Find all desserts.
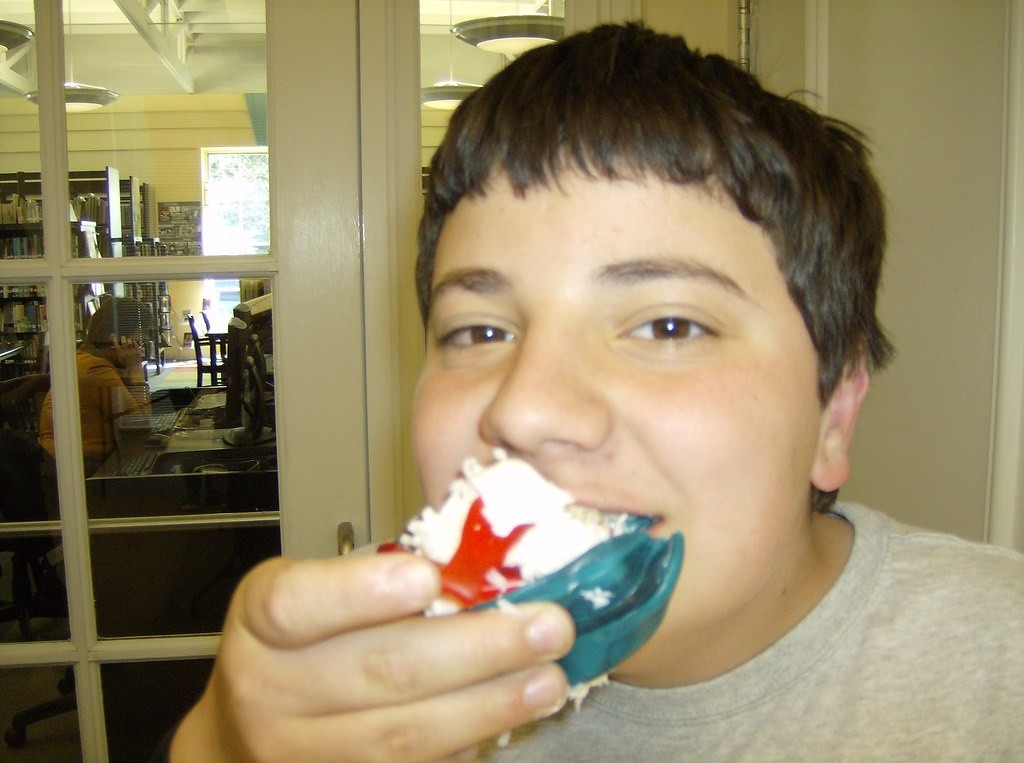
[368,447,685,702]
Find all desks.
[205,325,229,386]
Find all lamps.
[22,0,120,115]
[448,16,563,53]
[420,0,482,110]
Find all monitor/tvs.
[223,293,278,445]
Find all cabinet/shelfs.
[0,167,172,381]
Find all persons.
[20,297,151,599]
[151,21,1024,763]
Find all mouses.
[144,433,168,448]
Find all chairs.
[0,374,237,749]
[188,311,228,387]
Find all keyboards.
[117,453,160,477]
[149,410,183,436]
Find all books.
[0,287,83,331]
[0,234,79,259]
[0,335,38,364]
[0,192,146,229]
